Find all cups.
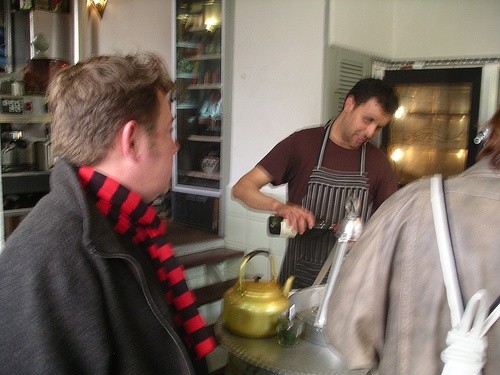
[275,313,304,348]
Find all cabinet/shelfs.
[0,1,227,237]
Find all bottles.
[266,216,341,239]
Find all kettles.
[221,249,294,339]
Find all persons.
[325,109,500,375]
[231,78,399,290]
[0,49,222,375]
